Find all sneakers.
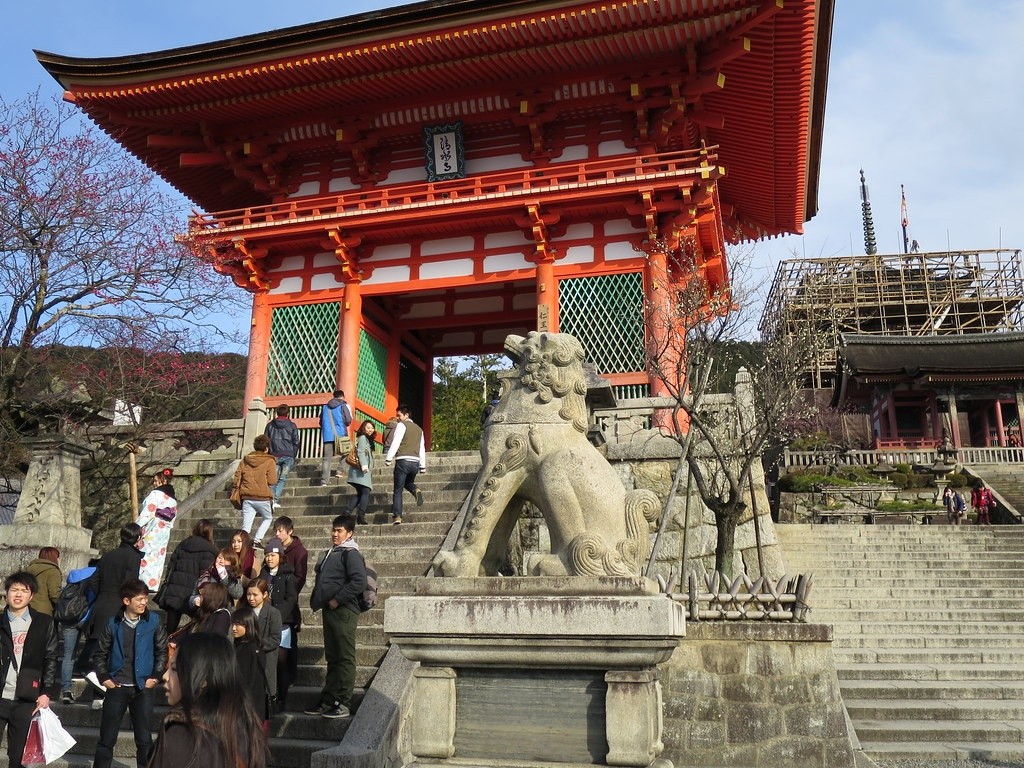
[252,543,265,551]
[304,700,352,718]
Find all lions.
[432,330,663,580]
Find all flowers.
[164,470,170,475]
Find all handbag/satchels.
[345,446,361,469]
[333,436,351,455]
[21,706,78,765]
[16,668,43,700]
[267,695,276,720]
[230,488,243,510]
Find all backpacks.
[341,547,378,611]
[55,580,90,625]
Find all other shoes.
[63,692,76,704]
[85,671,107,710]
[416,489,423,506]
[335,471,344,477]
[357,517,368,525]
[393,516,402,524]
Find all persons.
[135,468,178,593]
[304,515,367,718]
[319,390,352,486]
[146,632,276,768]
[233,404,300,551]
[943,486,963,525]
[0,515,308,768]
[971,482,997,525]
[343,420,377,525]
[382,405,426,524]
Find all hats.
[265,538,284,554]
